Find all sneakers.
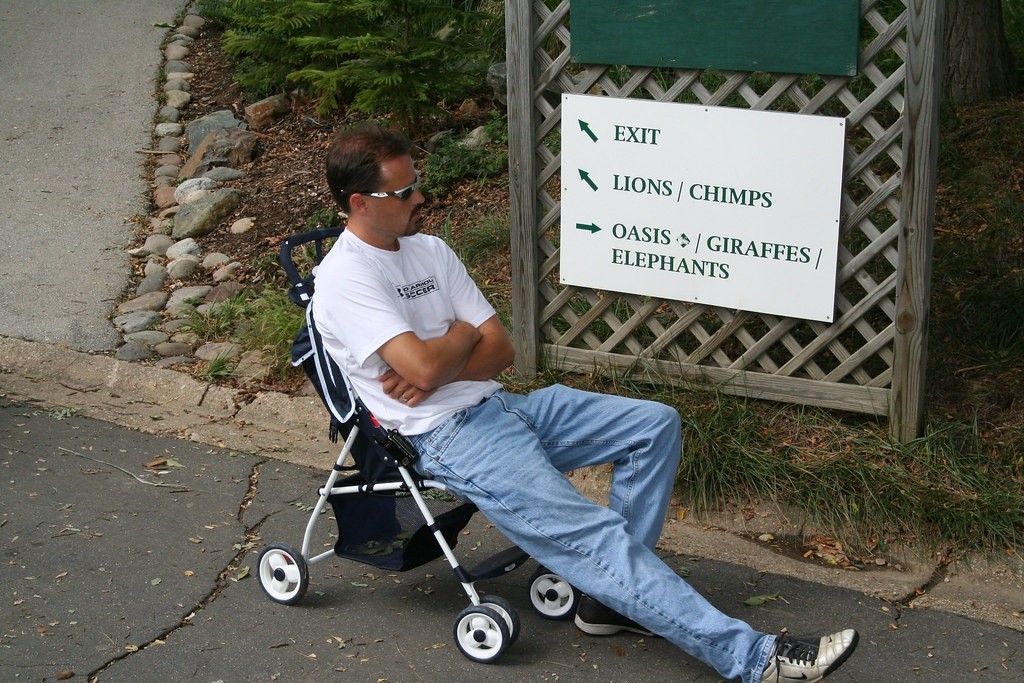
[573,594,656,636]
[759,628,860,683]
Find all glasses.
[355,174,421,202]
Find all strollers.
[257,228,579,663]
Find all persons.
[312,130,859,683]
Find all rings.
[402,395,407,401]
